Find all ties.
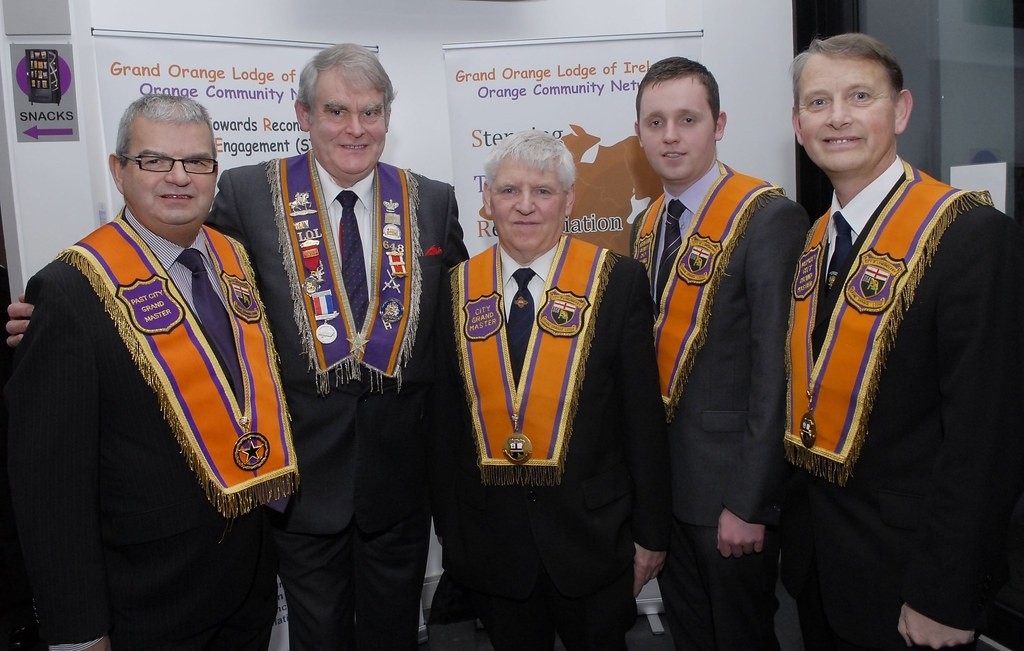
[507,267,534,392]
[815,212,852,297]
[335,190,369,332]
[656,200,686,316]
[176,250,245,414]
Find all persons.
[630,54,809,651]
[780,31,1024,651]
[0,95,299,650]
[6,40,472,651]
[415,130,670,650]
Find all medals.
[233,431,270,471]
[800,413,818,449]
[502,432,534,464]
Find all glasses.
[116,152,218,175]
